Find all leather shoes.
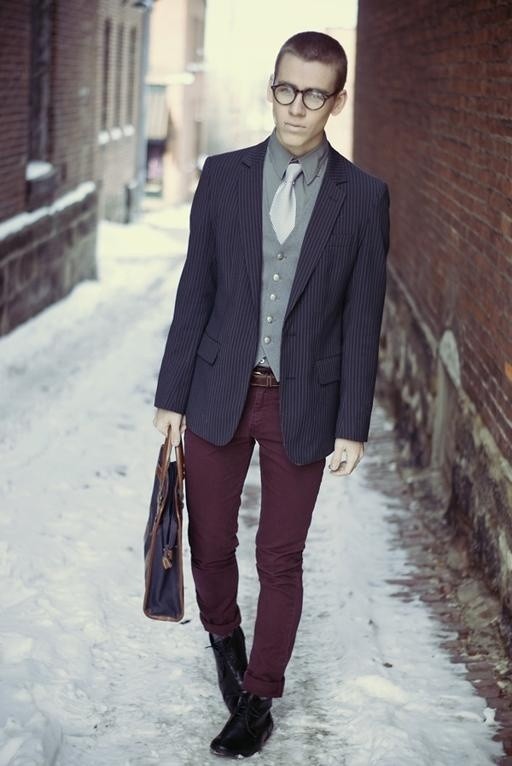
[204,625,248,716]
[209,691,274,759]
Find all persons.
[150,29,392,759]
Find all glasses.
[270,73,340,112]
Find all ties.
[268,163,303,245]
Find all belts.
[253,375,280,388]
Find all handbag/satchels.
[142,424,187,623]
